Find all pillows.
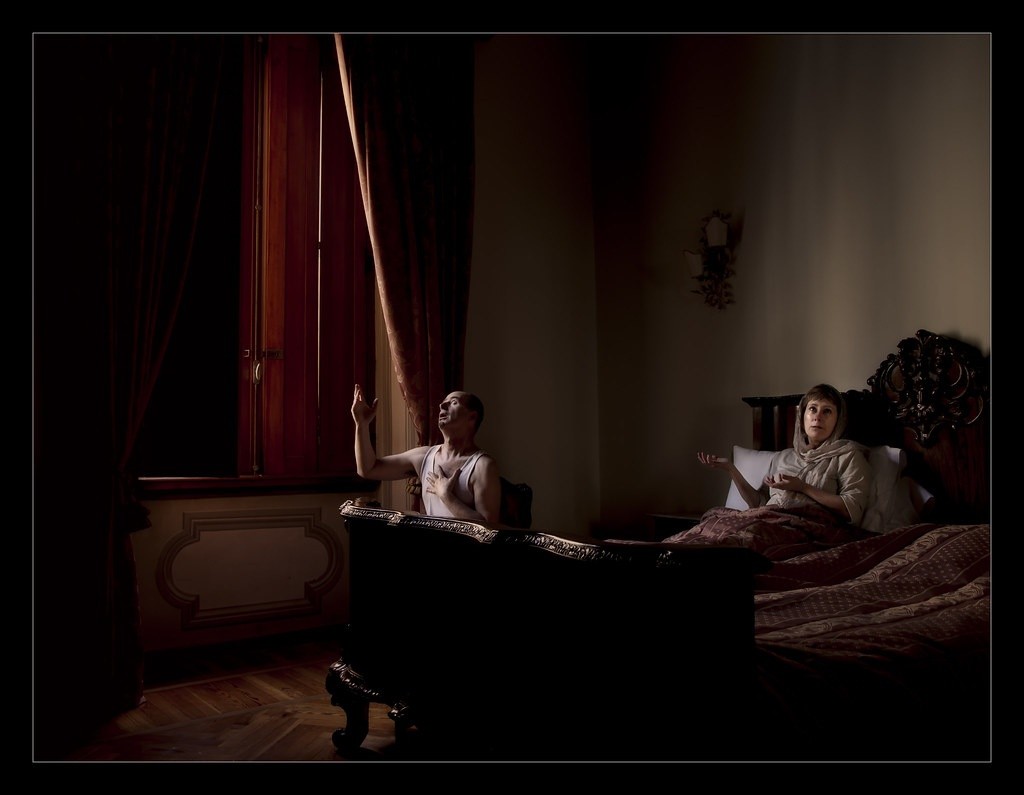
[724,444,936,534]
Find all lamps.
[681,209,734,311]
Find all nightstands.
[588,511,703,543]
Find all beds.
[325,328,990,762]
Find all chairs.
[499,476,533,528]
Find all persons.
[351,383,501,523]
[697,384,872,527]
